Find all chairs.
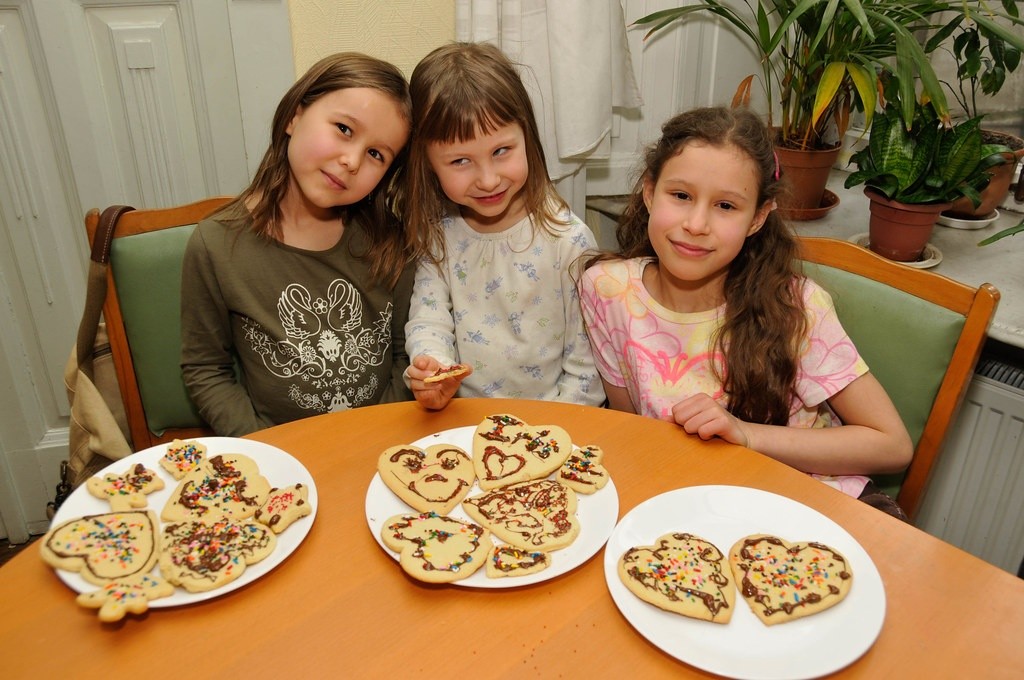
[84,197,240,453]
[797,234,1002,521]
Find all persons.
[400,39,614,407]
[175,50,421,436]
[575,104,917,518]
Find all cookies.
[41,363,853,628]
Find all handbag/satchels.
[45,205,138,527]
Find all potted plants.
[625,0,1024,222]
[844,102,1018,268]
[911,1,1023,230]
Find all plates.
[49,438,318,608]
[365,425,620,589]
[602,484,887,680]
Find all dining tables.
[0,397,1024,680]
